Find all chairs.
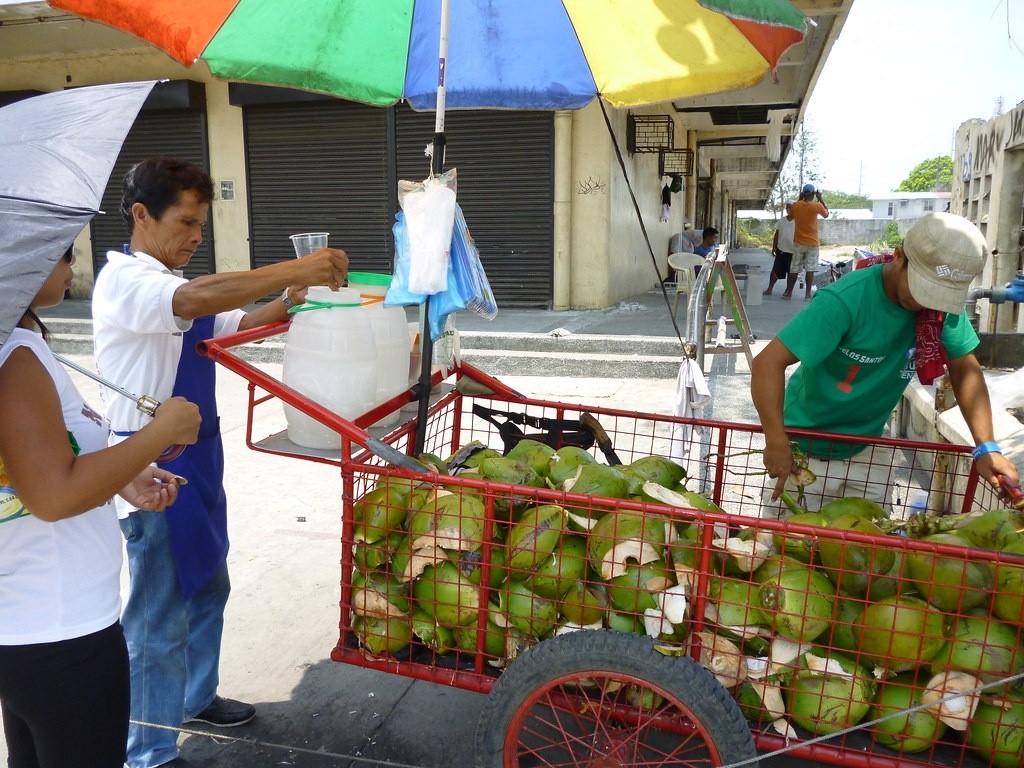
[667,252,725,320]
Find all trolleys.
[195,308,1024,768]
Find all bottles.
[280,285,411,449]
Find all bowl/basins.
[346,271,393,286]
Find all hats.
[803,184,814,194]
[904,210,989,315]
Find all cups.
[288,232,329,259]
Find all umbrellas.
[0,79,187,460]
[45,0,819,460]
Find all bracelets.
[283,287,294,308]
[972,442,1002,461]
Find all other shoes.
[763,290,772,294]
[783,293,791,299]
[805,296,812,301]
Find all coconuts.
[754,496,1024,768]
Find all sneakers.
[158,757,193,768]
[182,696,257,726]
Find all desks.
[733,266,762,306]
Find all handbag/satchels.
[472,404,594,456]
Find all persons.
[0,239,203,768]
[92,158,350,768]
[763,200,795,298]
[784,184,829,302]
[693,227,719,279]
[750,212,1021,549]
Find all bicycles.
[818,259,847,285]
